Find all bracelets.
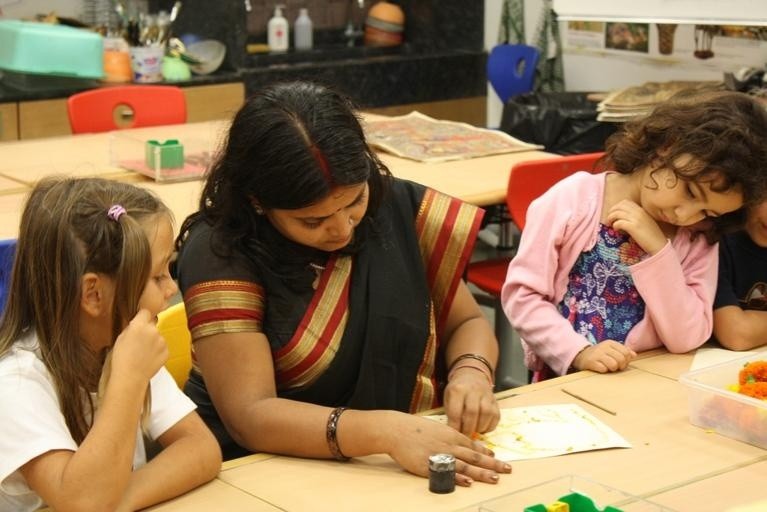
[325,405,352,462]
[447,353,494,376]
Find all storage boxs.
[677,346,767,452]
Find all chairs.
[68,85,187,136]
[155,304,192,390]
[463,152,615,300]
[484,45,540,104]
[0,239,18,319]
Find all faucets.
[342,0,364,46]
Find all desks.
[216,369,767,512]
[123,110,568,250]
[0,133,203,263]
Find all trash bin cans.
[497,87,621,157]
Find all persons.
[0,175,223,512]
[175,78,513,488]
[712,199,767,351]
[500,92,767,382]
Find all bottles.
[293,7,314,50]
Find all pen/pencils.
[562,387,618,415]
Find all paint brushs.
[102,1,183,45]
